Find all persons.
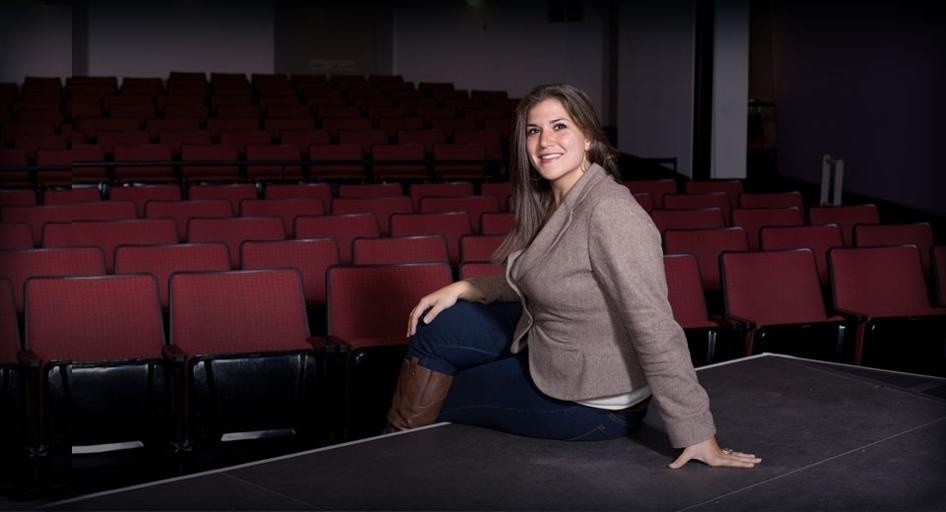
[386,82,762,471]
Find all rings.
[723,446,733,454]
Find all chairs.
[241,239,336,304]
[352,238,448,263]
[830,246,943,367]
[461,261,503,281]
[114,243,231,316]
[662,254,720,366]
[169,270,343,475]
[460,235,510,261]
[760,224,845,280]
[0,277,22,506]
[22,272,169,490]
[327,262,453,440]
[0,246,107,313]
[720,249,847,358]
[853,221,936,275]
[665,229,750,290]
